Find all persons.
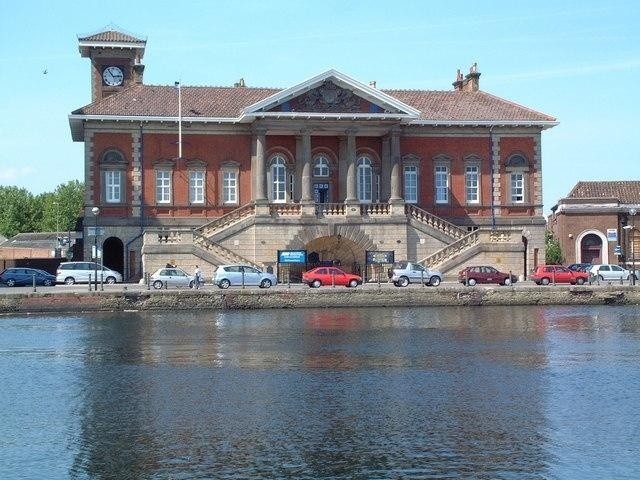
[191,265,201,290]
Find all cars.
[148,266,205,290]
[0,268,56,287]
[457,265,517,286]
[301,266,363,288]
[567,263,638,281]
[211,264,278,289]
[532,265,589,285]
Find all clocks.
[102,66,124,87]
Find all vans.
[56,261,123,285]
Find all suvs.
[388,261,444,287]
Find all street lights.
[91,206,101,290]
[629,209,637,285]
